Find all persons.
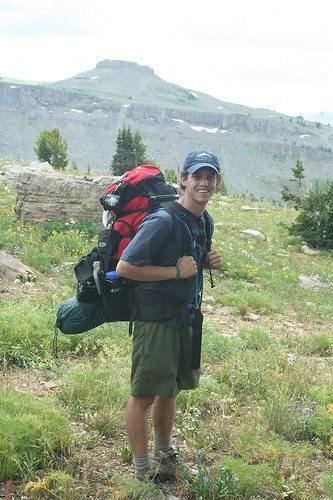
[115,150,222,500]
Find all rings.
[213,259,216,264]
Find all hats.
[183,150,220,175]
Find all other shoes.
[134,451,191,490]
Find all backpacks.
[98,165,180,260]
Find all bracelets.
[175,264,180,282]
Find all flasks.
[105,271,121,287]
